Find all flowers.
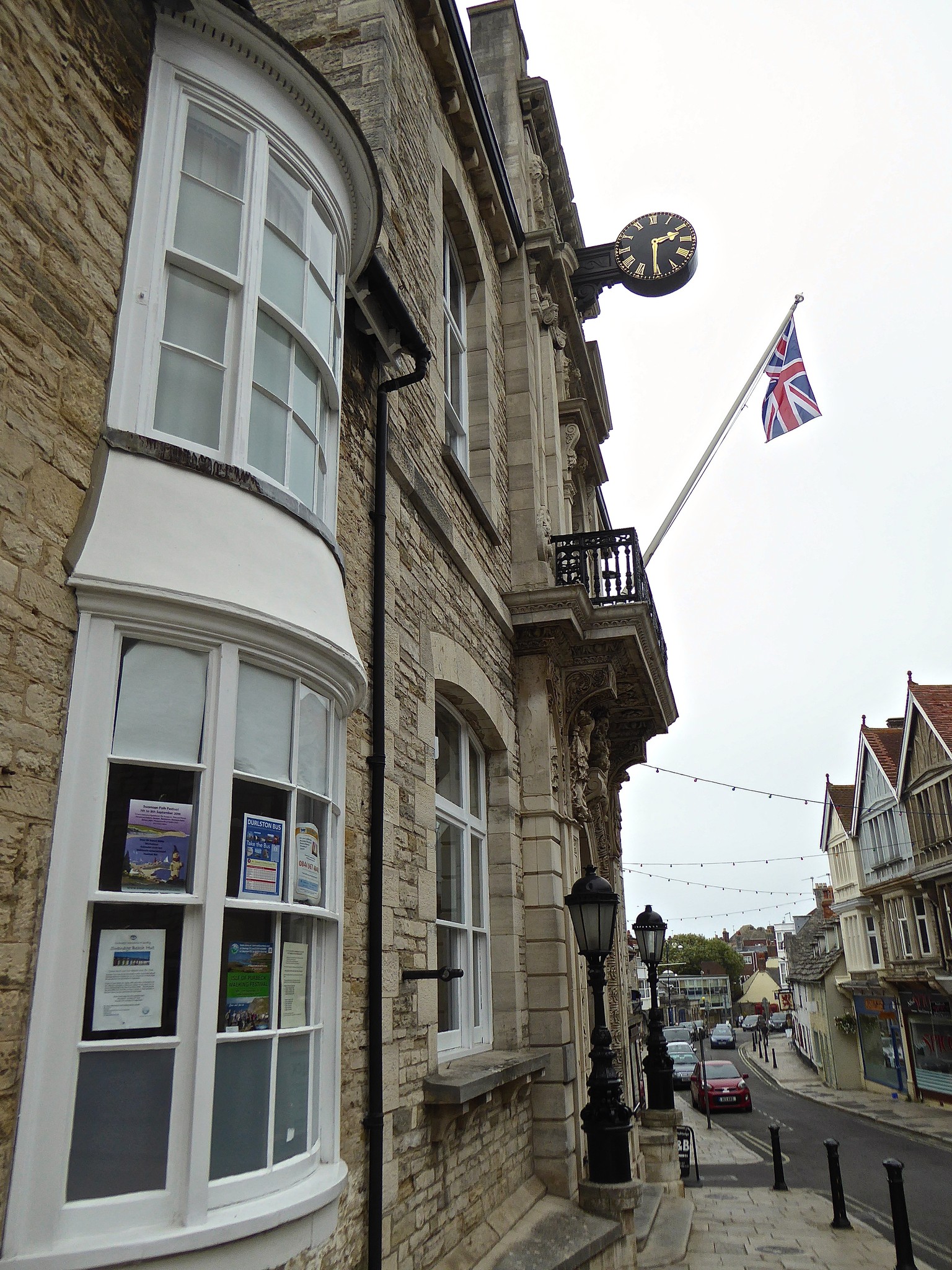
[833,1011,859,1036]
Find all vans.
[742,1014,766,1032]
[769,1013,792,1032]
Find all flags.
[762,317,822,443]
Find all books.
[92,800,321,1031]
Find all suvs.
[678,1021,700,1042]
[709,1023,736,1050]
[662,1025,697,1055]
[693,1020,709,1039]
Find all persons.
[637,1013,743,1051]
[761,1023,769,1046]
[770,1010,774,1020]
[760,1010,767,1024]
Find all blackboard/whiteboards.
[785,1028,792,1038]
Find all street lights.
[631,905,675,1111]
[664,938,683,1027]
[563,862,634,1184]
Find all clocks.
[613,211,700,300]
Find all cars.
[690,1060,752,1115]
[666,1041,697,1060]
[668,1052,703,1086]
